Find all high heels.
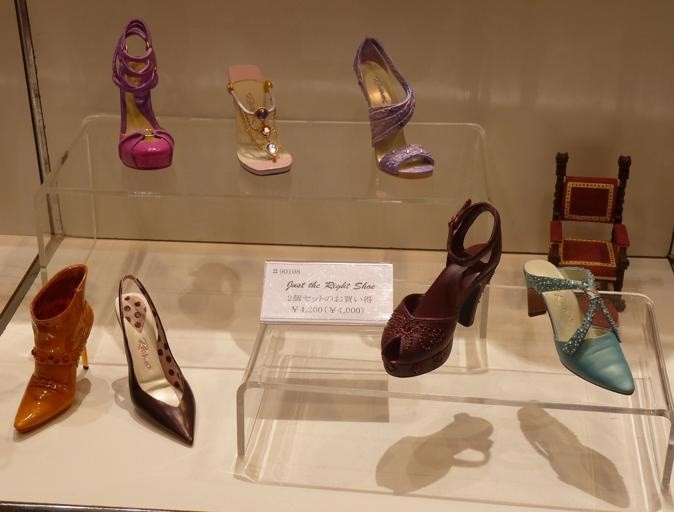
[227,65,292,175]
[112,18,174,169]
[353,36,434,177]
[524,259,635,395]
[13,264,93,432]
[115,275,195,445]
[379,199,502,378]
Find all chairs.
[545,151,631,312]
